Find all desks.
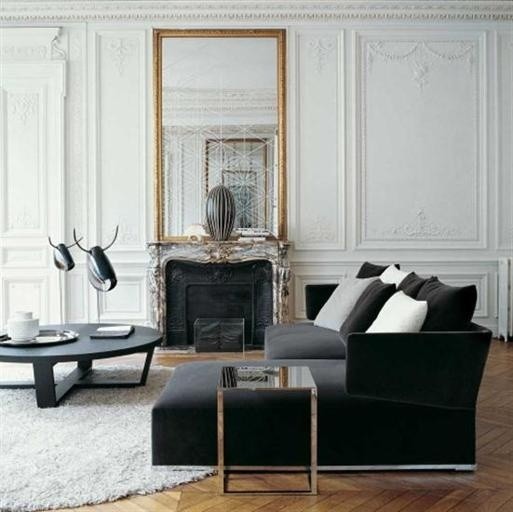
[214,365,318,496]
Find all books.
[238,230,270,240]
[89,326,133,339]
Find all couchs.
[150,321,493,474]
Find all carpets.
[0,365,217,512]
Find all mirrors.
[152,28,288,244]
[205,138,267,229]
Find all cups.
[13,311,32,319]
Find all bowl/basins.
[6,319,39,342]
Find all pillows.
[367,289,428,334]
[399,272,437,297]
[314,278,378,331]
[419,275,477,331]
[339,278,396,346]
[356,260,400,278]
[378,265,411,288]
